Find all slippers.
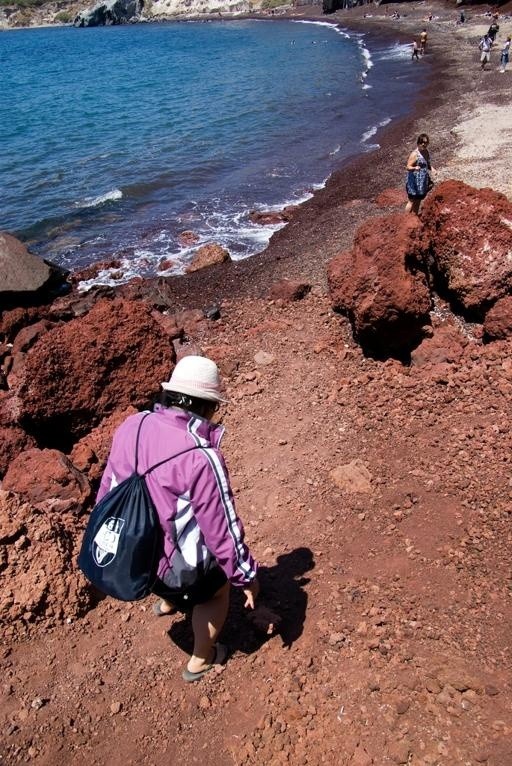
[182,643,229,684]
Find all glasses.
[420,141,429,144]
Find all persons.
[405,133,433,215]
[419,29,427,54]
[412,40,419,61]
[496,37,511,73]
[478,35,492,71]
[96,355,260,683]
[487,19,499,47]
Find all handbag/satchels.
[407,170,432,199]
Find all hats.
[160,354,231,408]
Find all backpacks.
[77,409,215,602]
